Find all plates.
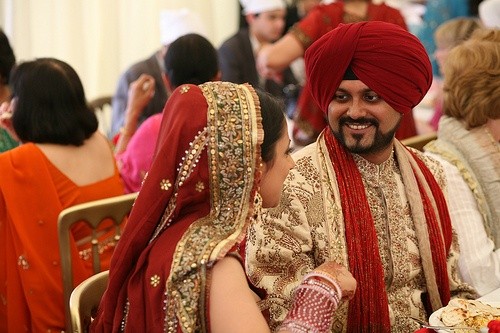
[429,307,492,333]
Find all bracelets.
[301,270,341,307]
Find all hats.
[239,0,286,14]
[304,19,433,114]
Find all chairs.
[57,190,141,333]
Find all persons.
[87,81,358,332]
[111,34,221,131]
[259,0,410,70]
[0,27,20,154]
[244,22,500,333]
[216,1,302,117]
[427,16,485,132]
[422,27,500,248]
[109,35,222,191]
[0,57,137,333]
